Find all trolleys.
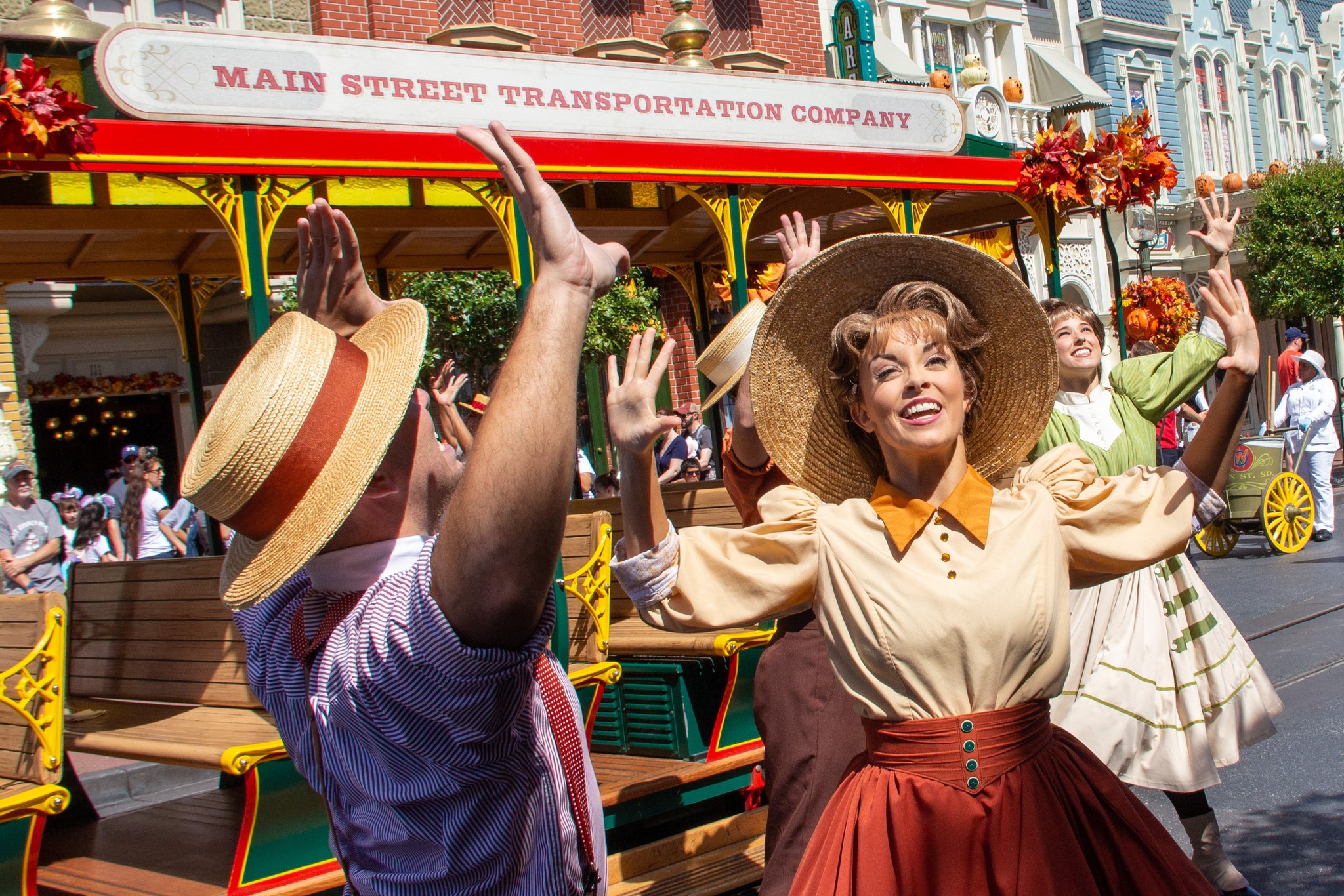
[1193,424,1314,559]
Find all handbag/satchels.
[1156,444,1164,466]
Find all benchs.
[0,480,779,896]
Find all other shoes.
[1331,476,1344,486]
[1299,533,1314,541]
[1222,886,1261,896]
[1314,529,1332,541]
[1190,558,1200,572]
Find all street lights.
[1117,191,1173,290]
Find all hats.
[695,297,768,412]
[1290,349,1327,377]
[179,298,429,612]
[459,393,491,415]
[121,445,140,460]
[1284,327,1308,342]
[2,462,33,482]
[749,231,1059,504]
[686,436,699,458]
[674,402,698,414]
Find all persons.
[695,207,866,896]
[1175,391,1212,450]
[0,463,69,599]
[50,442,234,584]
[569,445,596,501]
[672,402,717,482]
[1019,193,1284,896]
[427,358,493,463]
[1275,327,1344,486]
[183,120,627,896]
[1127,340,1201,575]
[655,407,688,484]
[594,469,620,497]
[1259,348,1340,542]
[680,458,702,482]
[604,219,1264,896]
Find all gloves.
[1259,426,1267,436]
[1298,418,1312,433]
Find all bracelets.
[24,581,35,591]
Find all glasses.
[125,455,138,463]
[147,467,164,473]
[686,470,700,476]
[677,413,690,418]
